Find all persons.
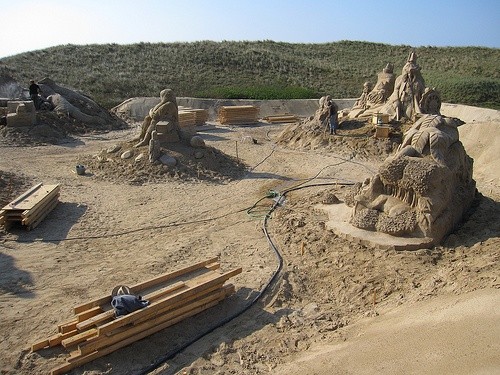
[382,53,428,122]
[132,90,181,148]
[28,81,43,110]
[149,131,160,162]
[326,103,338,135]
[368,61,396,105]
